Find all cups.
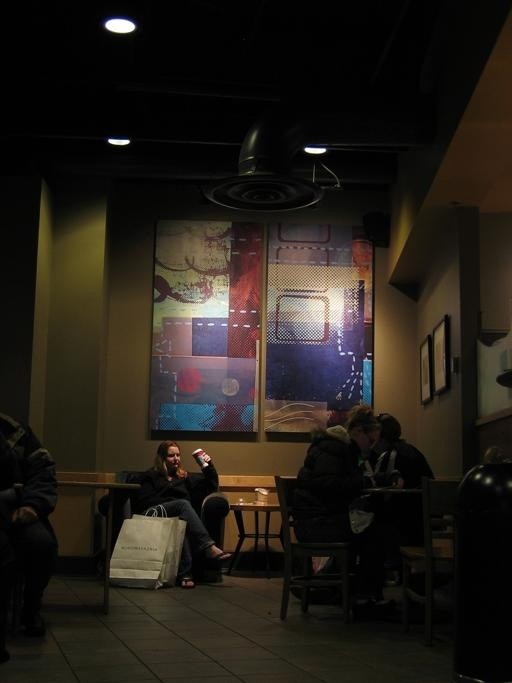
[191,449,209,470]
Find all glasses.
[365,433,375,447]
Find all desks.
[11,474,142,621]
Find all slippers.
[204,552,234,562]
[181,579,195,589]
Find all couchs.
[97,469,228,585]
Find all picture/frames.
[411,314,455,405]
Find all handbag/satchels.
[109,504,188,590]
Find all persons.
[289,404,441,618]
[134,438,233,589]
[0,408,60,648]
